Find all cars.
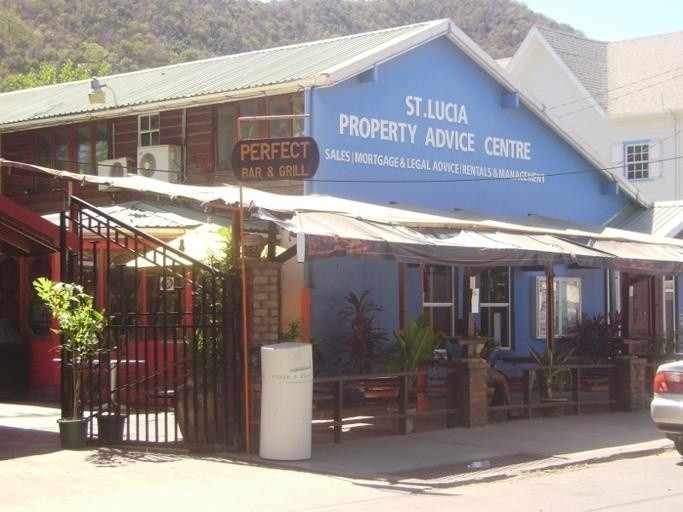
[648,359,683,456]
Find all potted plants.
[386,309,442,435]
[33,276,114,449]
[521,338,575,417]
[96,306,127,442]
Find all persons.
[462,328,518,419]
